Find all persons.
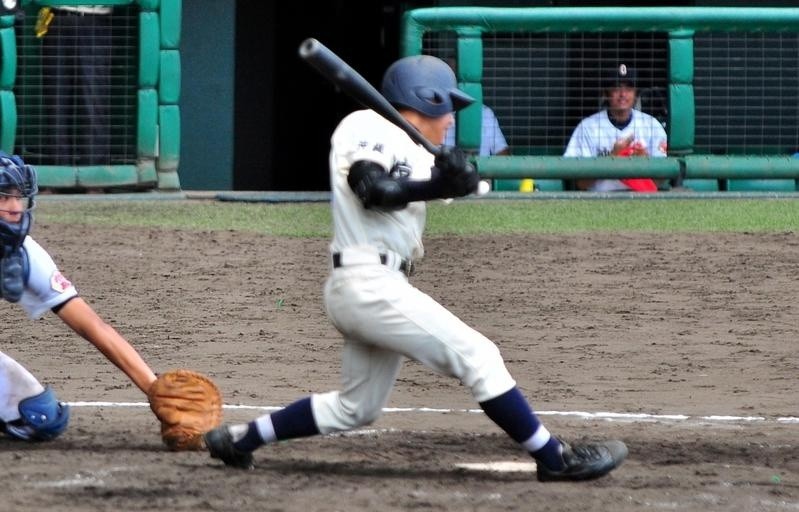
[38,4,114,194]
[443,55,511,156]
[563,63,667,191]
[202,54,628,481]
[0,155,222,449]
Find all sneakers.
[203,426,255,471]
[536,440,629,482]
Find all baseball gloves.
[147,370,223,451]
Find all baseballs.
[474,179,490,196]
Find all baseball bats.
[298,37,491,199]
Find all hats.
[603,63,637,89]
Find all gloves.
[434,145,479,198]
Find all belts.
[333,251,414,276]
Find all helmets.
[0,154,37,213]
[381,54,477,117]
